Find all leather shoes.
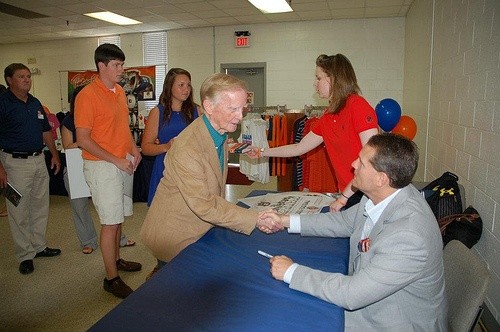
[19,260,34,275]
[35,246,61,257]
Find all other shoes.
[117,259,142,272]
[83,246,93,254]
[125,239,135,246]
[103,276,134,299]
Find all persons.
[246,54,380,212]
[140,73,284,267]
[259,133,448,332]
[73,43,143,300]
[61,86,136,254]
[142,67,201,205]
[0,63,61,274]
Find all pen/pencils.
[258,250,273,259]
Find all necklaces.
[175,106,180,115]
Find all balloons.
[392,115,417,141]
[374,98,401,133]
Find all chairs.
[443,240,490,332]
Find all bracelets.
[259,146,265,159]
[341,192,350,200]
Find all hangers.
[245,105,328,119]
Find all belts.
[0,148,43,156]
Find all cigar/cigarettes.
[258,249,275,260]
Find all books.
[0,181,23,208]
[227,141,251,154]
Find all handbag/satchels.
[418,171,462,219]
[437,206,483,250]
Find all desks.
[42,148,155,203]
[87,191,350,332]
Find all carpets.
[226,168,256,186]
